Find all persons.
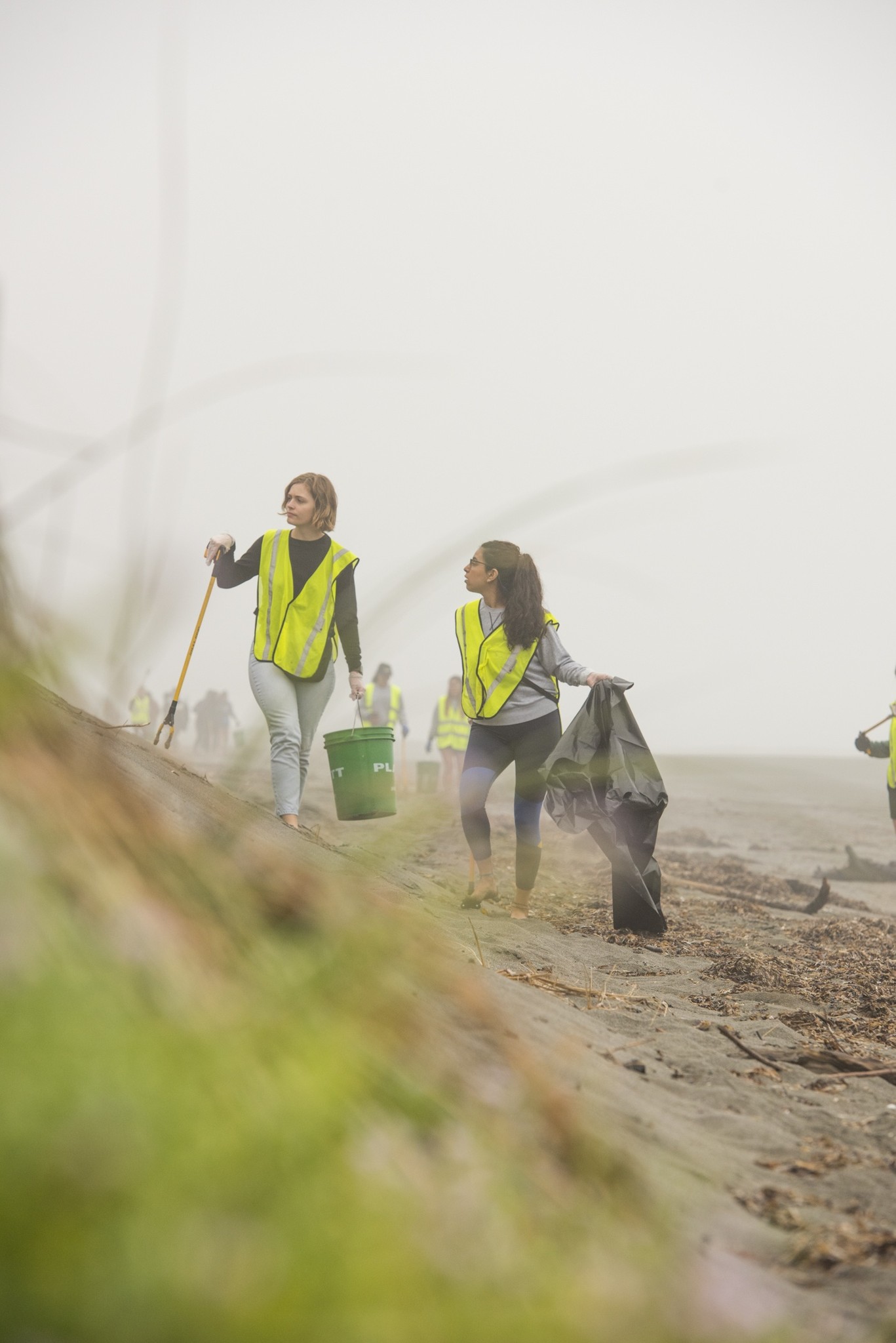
[424,674,472,813]
[453,537,615,923]
[354,664,409,738]
[205,472,367,834]
[854,664,896,824]
[130,684,241,766]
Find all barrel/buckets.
[417,754,441,791]
[232,723,246,748]
[323,693,397,821]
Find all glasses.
[469,558,487,568]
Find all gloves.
[854,735,870,751]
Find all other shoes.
[461,871,499,908]
